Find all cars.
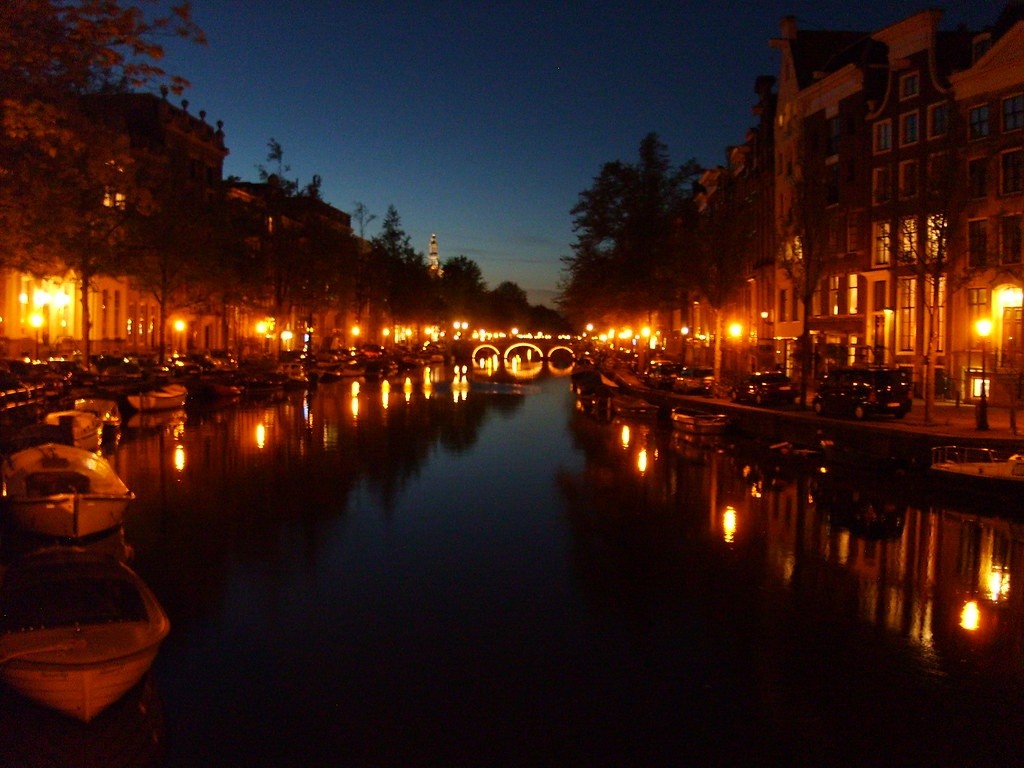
[643,361,677,387]
[673,366,714,394]
[728,371,796,408]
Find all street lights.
[975,317,991,430]
[32,314,41,360]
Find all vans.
[813,365,911,418]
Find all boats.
[1,541,170,722]
[34,395,124,454]
[570,351,618,393]
[189,350,455,403]
[0,442,170,544]
[925,445,1024,512]
[610,391,659,420]
[669,405,727,434]
[128,381,188,410]
